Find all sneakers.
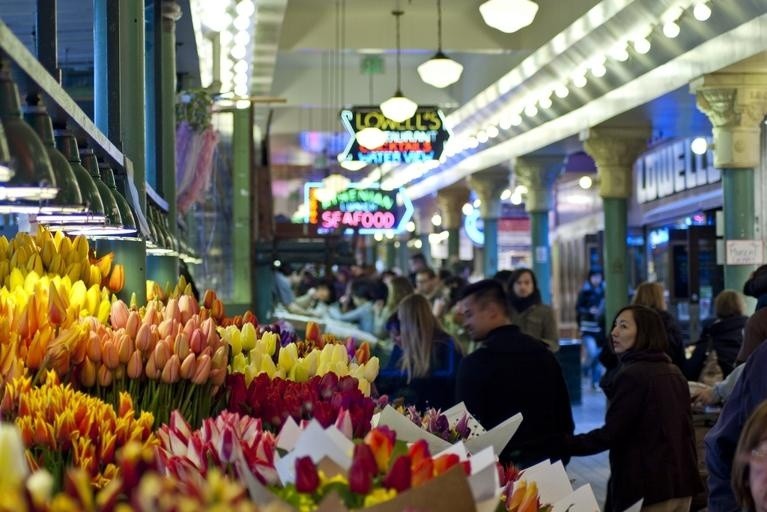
[582,365,602,394]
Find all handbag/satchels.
[699,347,725,387]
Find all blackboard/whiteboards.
[584,242,603,282]
[624,245,648,289]
[668,240,690,305]
[688,224,724,287]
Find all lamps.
[0,54,202,266]
[336,0,541,170]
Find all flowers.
[0,223,573,510]
